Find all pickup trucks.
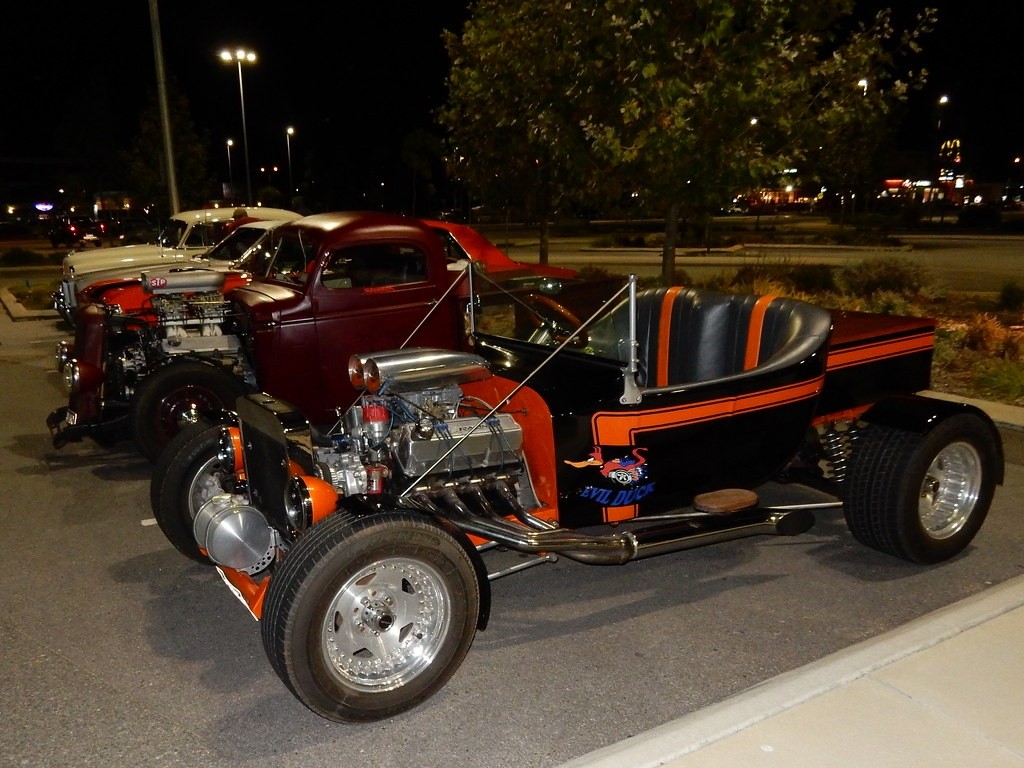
[42,206,625,467]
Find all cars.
[40,211,107,249]
[45,205,581,341]
[145,256,1007,726]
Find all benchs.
[609,286,835,412]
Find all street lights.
[226,139,235,205]
[219,47,259,206]
[285,126,296,207]
